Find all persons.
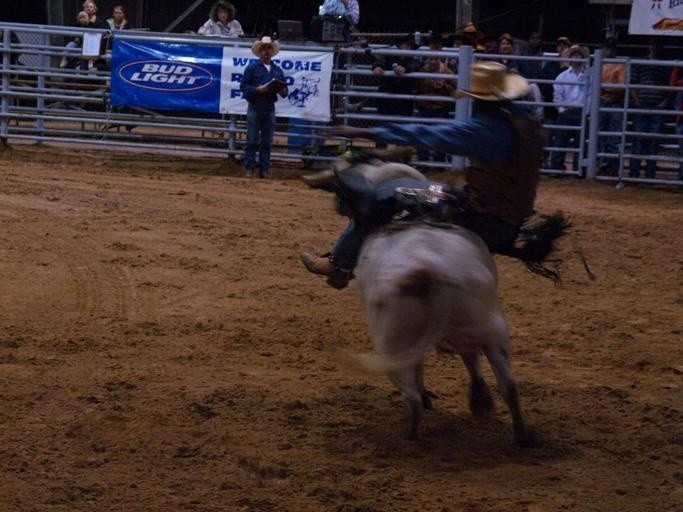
[372,21,683,191]
[301,61,546,289]
[60,1,136,114]
[199,2,243,38]
[1,30,22,151]
[320,1,359,24]
[241,36,288,180]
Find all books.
[264,78,286,96]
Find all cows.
[295,144,533,463]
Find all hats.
[250,36,281,57]
[560,44,590,67]
[497,33,513,47]
[556,37,572,46]
[455,21,485,40]
[452,61,531,102]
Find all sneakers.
[259,170,273,180]
[244,169,253,177]
[300,250,355,290]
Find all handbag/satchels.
[303,14,352,43]
